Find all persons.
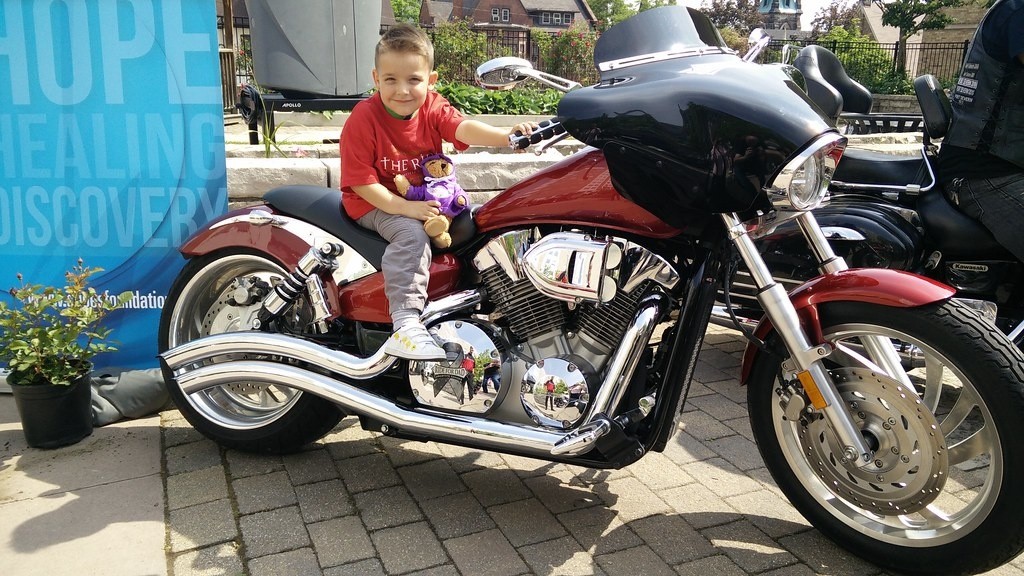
[939,0,1024,264]
[340,25,539,362]
[460,346,474,404]
[482,359,500,393]
[735,136,766,186]
[544,376,555,411]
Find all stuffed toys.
[394,154,468,247]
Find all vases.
[6,360,94,452]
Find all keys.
[951,190,959,205]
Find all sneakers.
[385,323,447,361]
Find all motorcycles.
[155,2,1024,576]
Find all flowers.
[0,256,133,386]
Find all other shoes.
[552,409,555,411]
[545,408,547,410]
